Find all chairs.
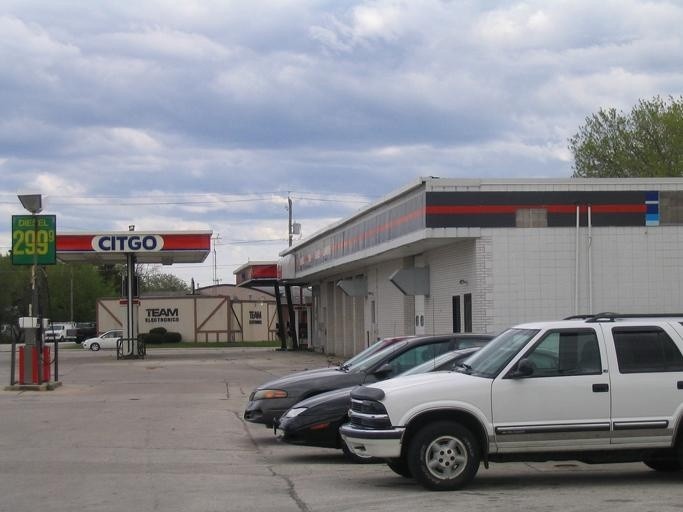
[576,340,600,374]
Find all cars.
[44,321,124,351]
